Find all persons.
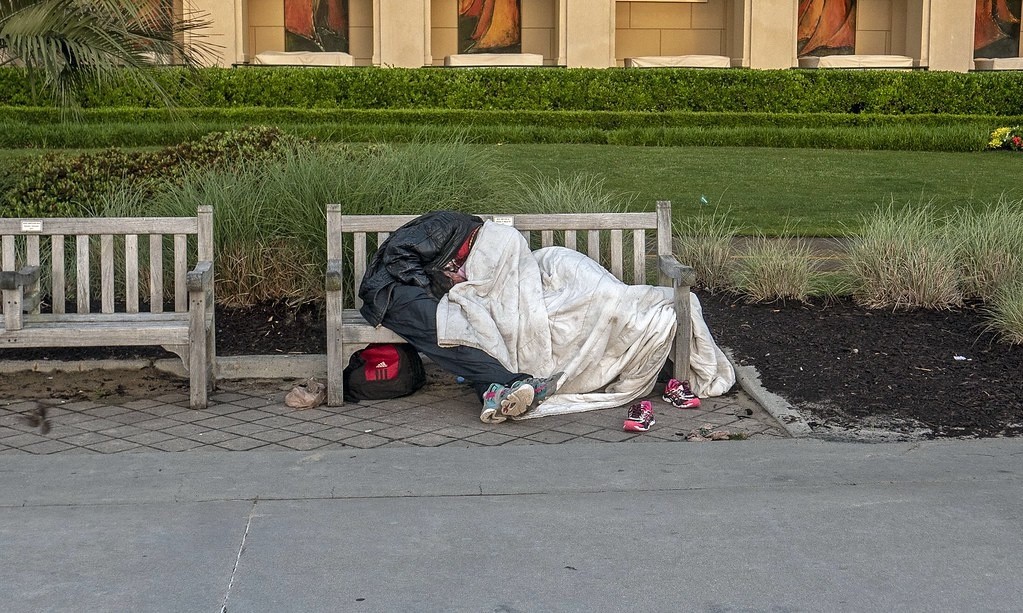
[359,208,570,423]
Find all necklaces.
[469,224,484,251]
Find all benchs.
[324,195,697,407]
[0,207,222,408]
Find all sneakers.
[479,382,534,424]
[622,400,657,431]
[510,371,570,421]
[663,378,703,410]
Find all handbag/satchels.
[342,342,427,404]
